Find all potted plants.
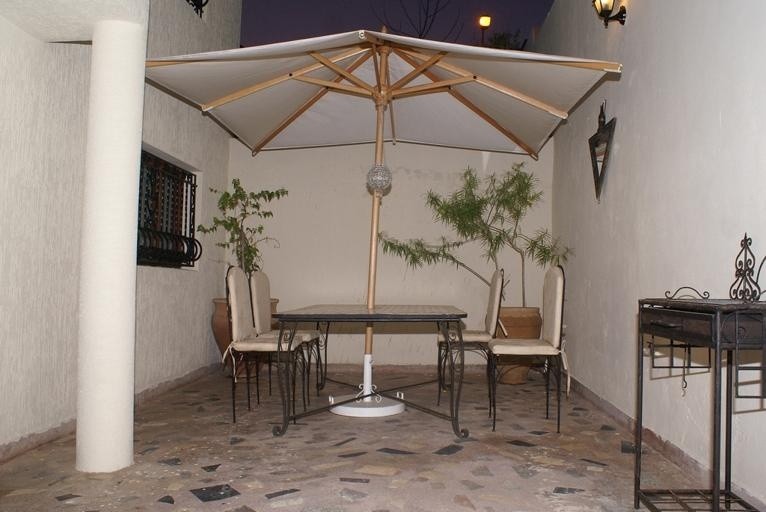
[375,163,576,385]
[196,178,290,378]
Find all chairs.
[486,264,566,434]
[437,268,505,406]
[226,265,322,426]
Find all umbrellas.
[143,25,622,403]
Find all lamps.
[592,0,626,26]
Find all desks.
[271,302,469,439]
[633,231,766,511]
